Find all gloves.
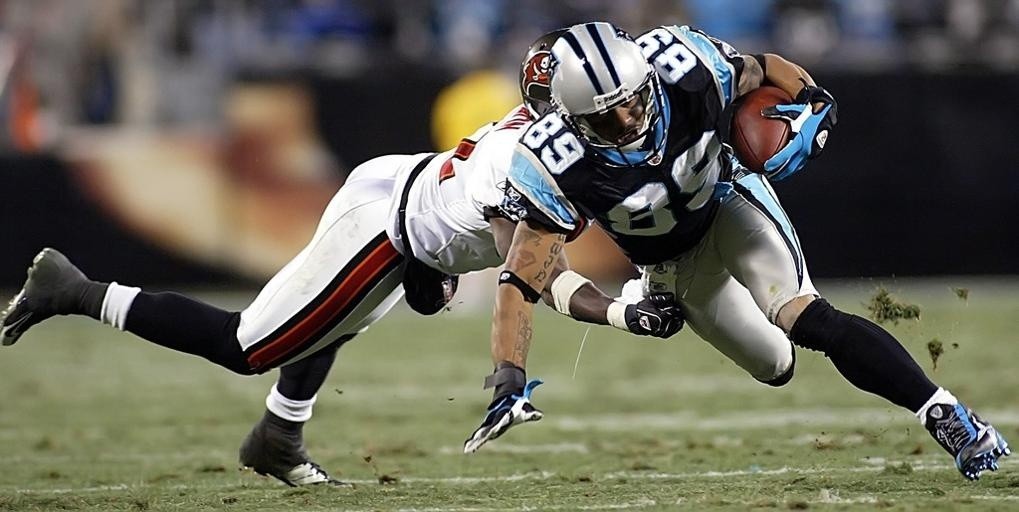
[759,76,840,184]
[624,290,686,341]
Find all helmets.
[517,19,669,173]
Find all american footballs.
[733,86,793,174]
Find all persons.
[0,23,686,484]
[455,14,1012,485]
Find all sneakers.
[0,244,114,349]
[237,405,334,490]
[923,400,1013,484]
[460,360,545,456]
